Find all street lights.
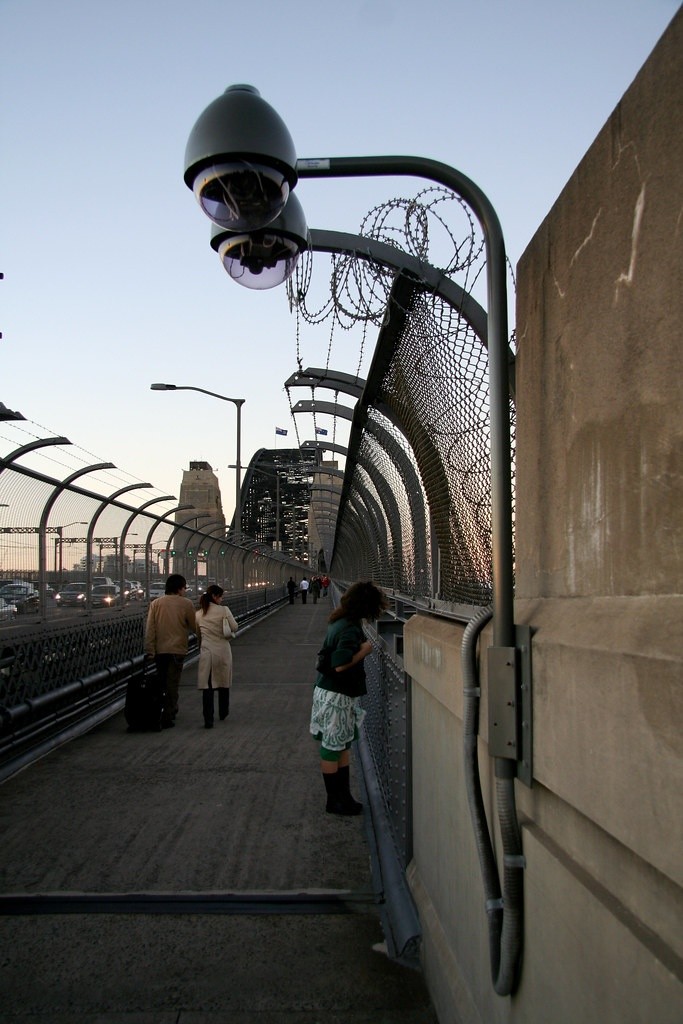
[150,540,169,576]
[257,499,296,558]
[228,465,280,553]
[150,382,245,539]
[59,521,89,570]
[114,533,138,578]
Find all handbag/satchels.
[223,605,235,641]
[315,624,365,684]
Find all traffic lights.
[171,549,225,566]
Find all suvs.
[92,576,114,586]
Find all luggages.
[123,653,164,732]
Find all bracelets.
[355,652,364,660]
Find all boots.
[322,765,364,816]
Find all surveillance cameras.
[184,84,300,233]
[210,191,307,291]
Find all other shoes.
[161,703,180,729]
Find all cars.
[55,583,93,607]
[0,584,40,616]
[109,578,166,602]
[32,582,55,600]
[81,584,121,609]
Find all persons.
[143,574,195,730]
[300,577,309,604]
[195,585,238,729]
[309,574,330,604]
[287,577,296,604]
[309,580,387,816]
